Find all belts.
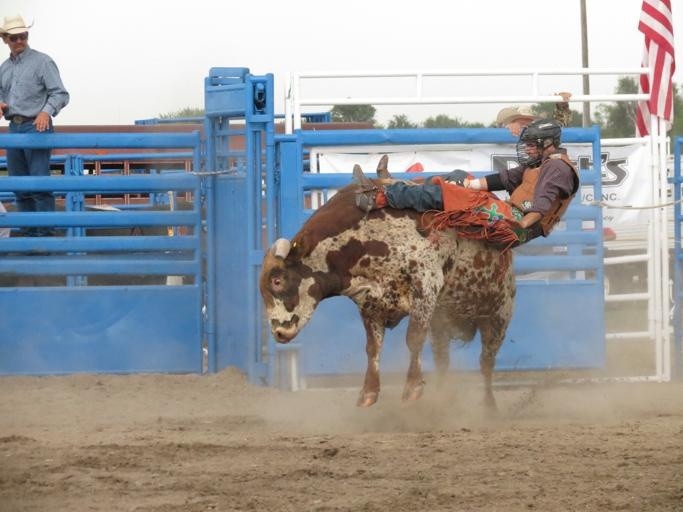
[9,115,34,125]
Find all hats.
[0,13,36,35]
[495,105,537,126]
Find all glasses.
[8,34,27,42]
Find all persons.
[496,109,541,139]
[539,89,581,128]
[350,117,579,255]
[0,10,69,260]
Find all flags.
[636,1,677,150]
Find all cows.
[259,177,516,412]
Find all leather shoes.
[375,154,392,179]
[351,163,379,213]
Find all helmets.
[523,118,563,149]
